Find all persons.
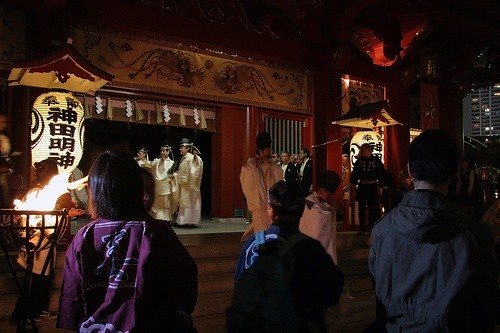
[445,148,480,201]
[130,139,207,230]
[239,132,345,264]
[228,182,344,332]
[341,143,388,235]
[369,130,499,332]
[0,114,15,210]
[12,158,86,331]
[57,150,198,333]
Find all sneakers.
[9,313,32,324]
[34,310,57,321]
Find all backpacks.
[227,230,305,333]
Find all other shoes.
[368,231,372,235]
[356,230,364,234]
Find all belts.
[360,179,377,183]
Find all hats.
[318,170,341,193]
[161,144,170,149]
[257,133,271,149]
[180,137,192,146]
